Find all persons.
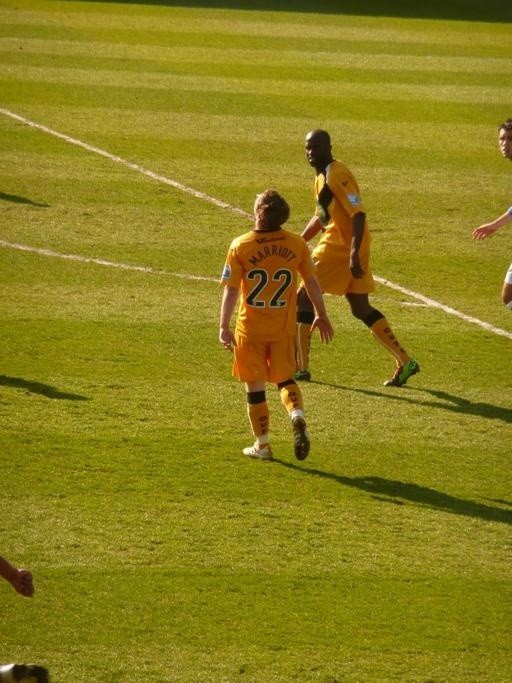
[0,556,52,683]
[218,189,334,461]
[471,118,512,310]
[296,128,420,386]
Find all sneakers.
[383,357,420,387]
[243,443,274,460]
[0,662,49,682]
[295,370,311,382]
[292,416,310,460]
